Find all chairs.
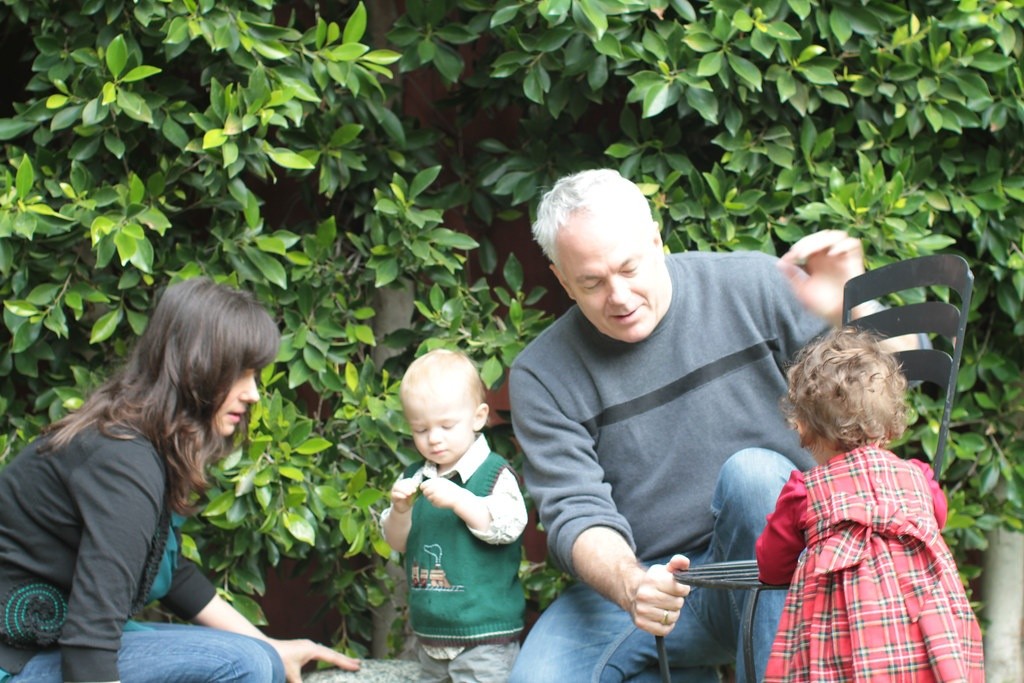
[643,251,980,683]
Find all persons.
[756,327,985,683]
[379,349,528,683]
[507,169,933,683]
[0,279,361,683]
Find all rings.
[662,610,670,625]
